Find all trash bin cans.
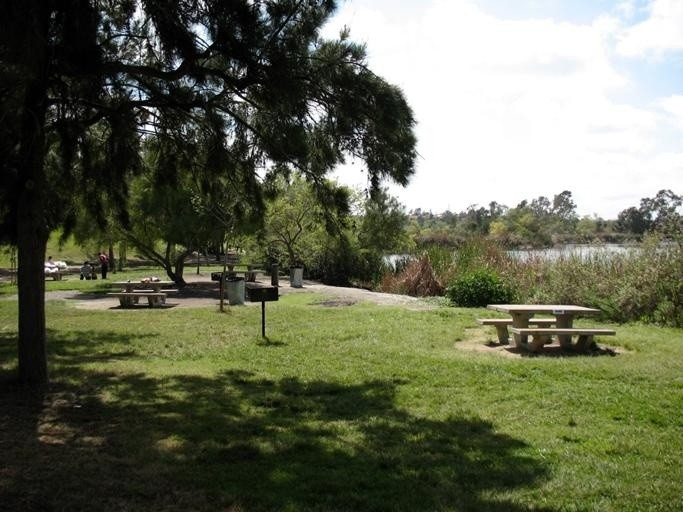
[290,265,304,288]
[225,276,245,304]
[271,264,279,286]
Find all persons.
[90,264,96,280]
[78,261,91,279]
[47,256,53,265]
[97,252,106,279]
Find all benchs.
[216,270,266,282]
[106,289,178,307]
[475,318,616,355]
[44,271,71,281]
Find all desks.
[486,304,601,349]
[45,267,65,280]
[220,264,264,281]
[111,281,175,307]
